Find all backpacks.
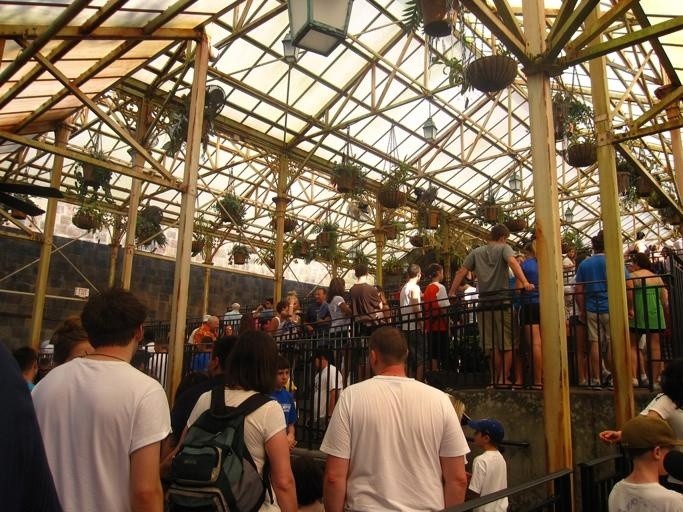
[165,382,277,512]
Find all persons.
[303,265,391,385]
[441,386,471,426]
[399,224,536,390]
[292,453,325,509]
[3,287,173,510]
[608,415,681,509]
[514,233,681,391]
[188,291,302,351]
[313,346,344,446]
[599,359,681,493]
[470,416,509,509]
[161,330,298,509]
[319,325,471,509]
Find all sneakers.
[602,371,611,384]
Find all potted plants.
[550,68,598,166]
[499,199,527,232]
[443,16,517,93]
[72,107,214,255]
[561,229,583,252]
[403,0,463,37]
[616,144,681,236]
[217,127,496,283]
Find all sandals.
[638,378,649,388]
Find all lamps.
[423,38,438,142]
[286,2,352,56]
[565,201,573,223]
[282,30,298,63]
[509,153,521,192]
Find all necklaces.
[81,350,125,361]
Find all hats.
[622,415,683,449]
[468,418,504,443]
[203,315,210,322]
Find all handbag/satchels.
[282,321,293,332]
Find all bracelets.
[328,416,331,418]
[382,303,388,305]
